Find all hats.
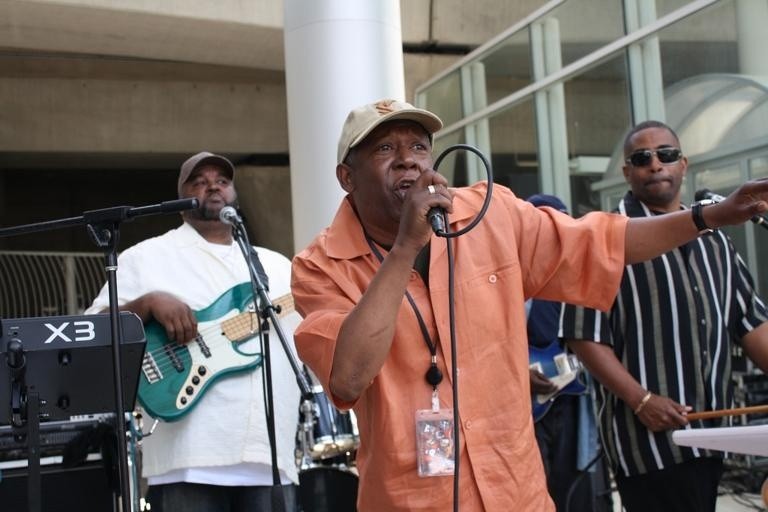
[335,97,444,168]
[525,192,570,215]
[176,150,236,196]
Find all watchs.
[691,198,720,236]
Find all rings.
[428,185,436,195]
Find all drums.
[300,384,361,462]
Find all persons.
[523,193,613,512]
[290,101,768,512]
[84,152,304,512]
[555,120,768,512]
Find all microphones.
[218,205,242,227]
[426,204,444,237]
[693,188,768,228]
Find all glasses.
[623,143,685,168]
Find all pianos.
[0,312,146,416]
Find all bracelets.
[633,391,651,415]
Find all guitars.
[529,341,593,424]
[134,282,295,422]
